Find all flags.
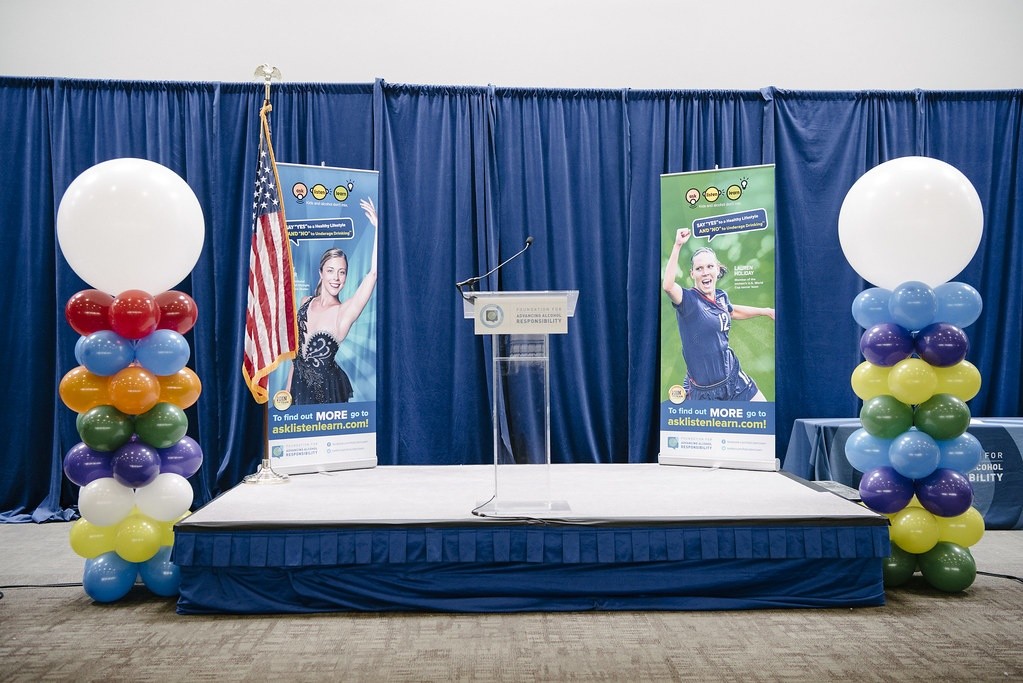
[241,103,298,405]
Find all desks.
[781,418,1023,530]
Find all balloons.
[57,159,205,297]
[845,427,982,478]
[882,540,976,592]
[851,282,983,334]
[859,323,969,368]
[859,468,973,517]
[75,405,188,451]
[851,359,982,406]
[859,494,984,553]
[77,473,194,525]
[74,330,190,376]
[60,363,202,414]
[67,291,198,336]
[70,509,193,601]
[63,436,203,489]
[860,394,971,441]
[838,157,983,291]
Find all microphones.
[455,237,534,288]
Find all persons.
[661,228,776,402]
[284,197,377,403]
